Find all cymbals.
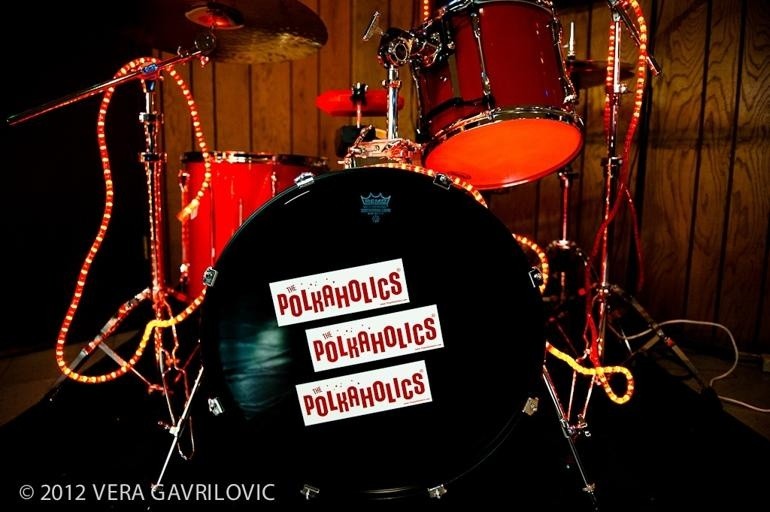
[561,61,635,89]
[148,0,328,64]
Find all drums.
[411,2,585,194]
[180,151,329,304]
[344,138,423,166]
[202,164,546,503]
